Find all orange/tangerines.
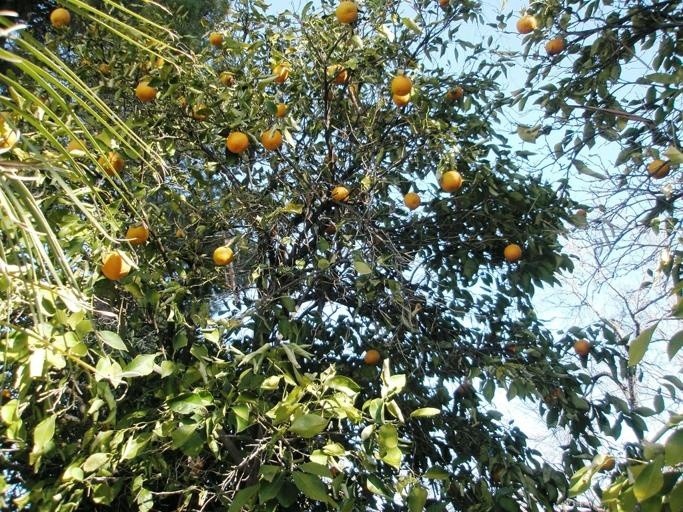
[0,1,671,472]
